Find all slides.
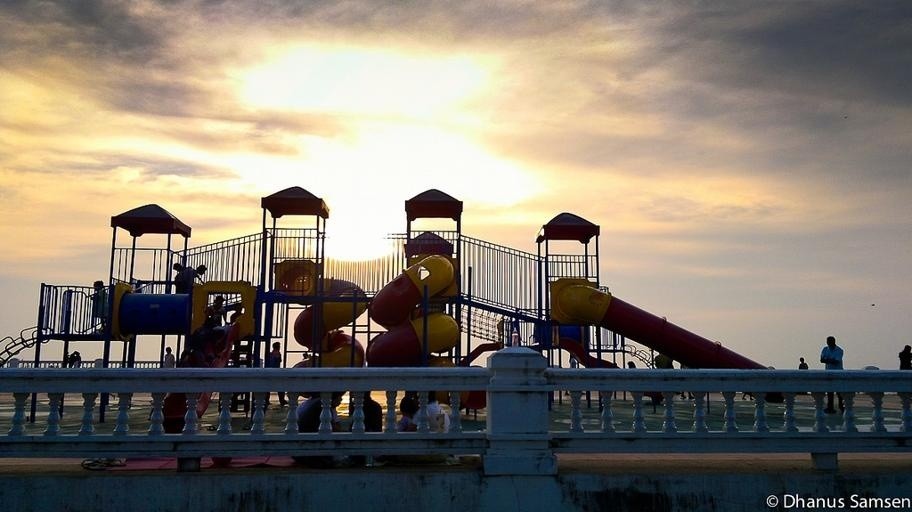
[163,323,238,425]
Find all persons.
[742,393,754,400]
[799,358,808,395]
[820,337,844,414]
[565,353,585,395]
[680,364,694,399]
[899,345,912,370]
